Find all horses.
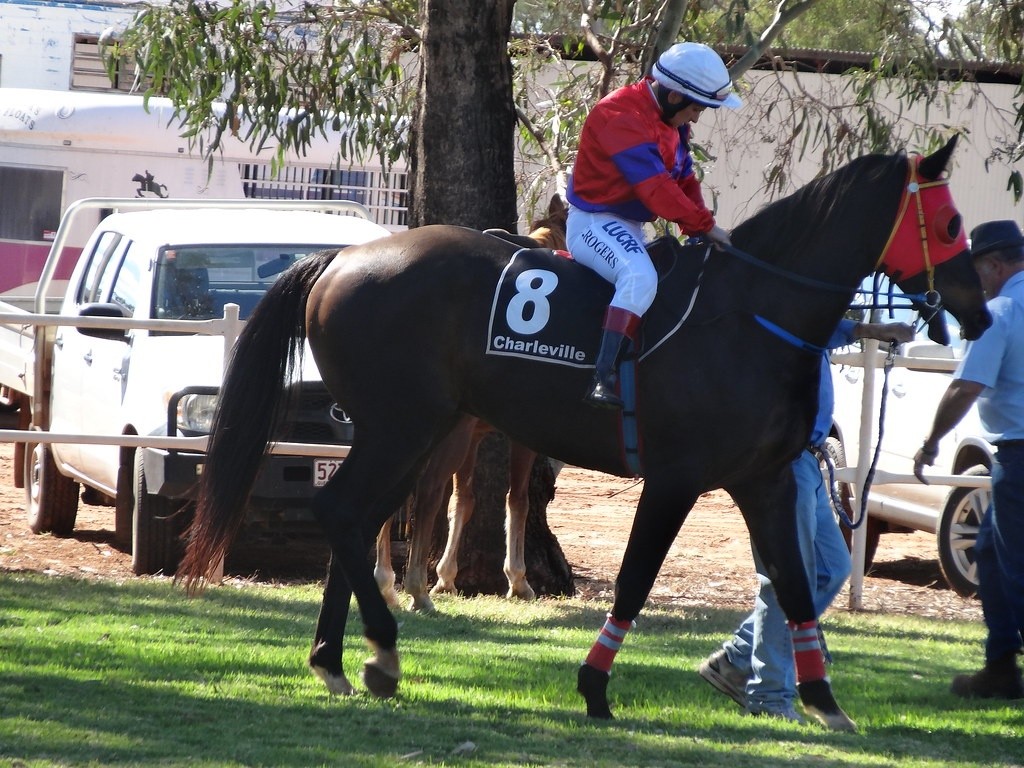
[153,133,993,730]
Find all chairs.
[166,269,219,317]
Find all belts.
[805,444,825,463]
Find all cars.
[806,236,1000,604]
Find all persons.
[565,42,745,416]
[913,220,1024,700]
[698,321,913,728]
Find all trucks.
[0,195,393,577]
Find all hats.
[970,220,1024,257]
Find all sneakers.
[765,709,806,726]
[951,667,1024,699]
[699,649,753,708]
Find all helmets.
[652,42,741,108]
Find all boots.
[585,305,641,411]
